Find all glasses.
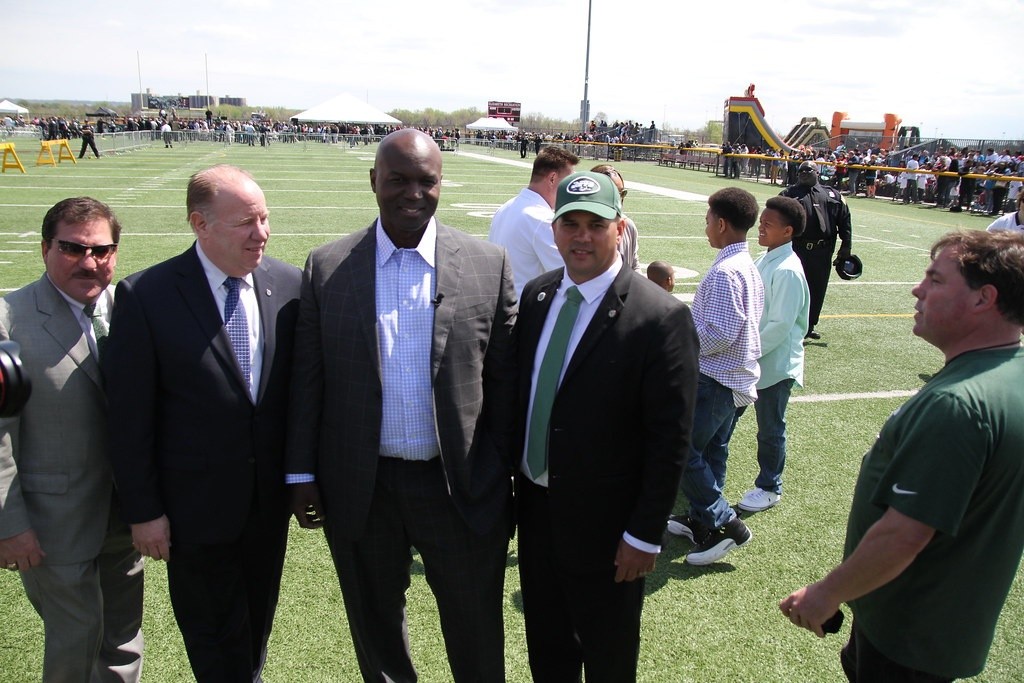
[618,189,628,198]
[48,236,118,262]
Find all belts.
[796,242,828,250]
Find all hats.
[549,170,622,223]
[836,255,863,281]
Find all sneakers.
[738,487,781,511]
[667,510,706,545]
[686,512,752,566]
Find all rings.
[6,563,17,571]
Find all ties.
[526,285,584,481]
[809,187,826,232]
[84,302,107,377]
[223,277,251,392]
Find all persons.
[506,171,701,683]
[466,120,656,158]
[778,161,852,339]
[647,261,675,292]
[669,139,1024,218]
[667,187,764,565]
[779,229,1024,683]
[986,188,1024,233]
[488,146,581,309]
[0,105,285,159]
[285,122,461,149]
[591,164,642,275]
[285,128,520,683]
[0,196,144,683]
[737,194,810,512]
[104,163,304,683]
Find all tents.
[86,107,119,133]
[0,100,29,126]
[466,117,518,144]
[289,93,402,142]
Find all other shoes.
[809,330,820,339]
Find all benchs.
[655,152,725,173]
